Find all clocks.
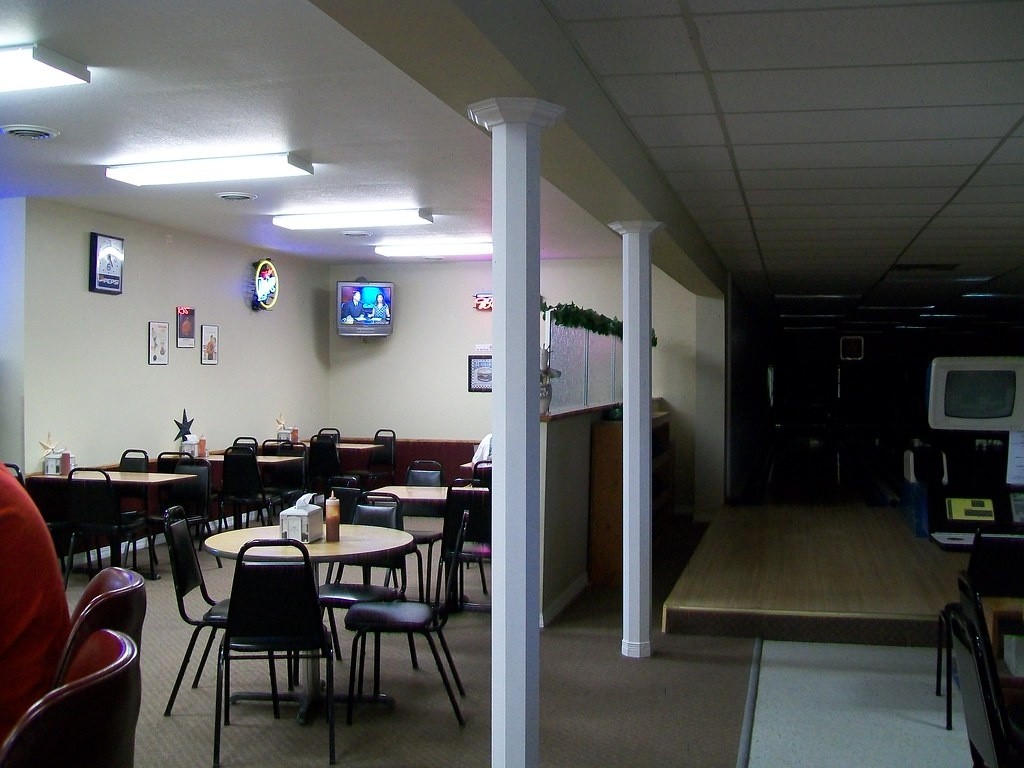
[90,231,124,295]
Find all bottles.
[70,463,74,474]
[325,490,340,542]
[298,437,301,443]
[75,463,79,474]
[199,433,206,457]
[292,425,298,443]
[60,445,70,475]
[206,448,210,457]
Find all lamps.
[0,44,91,92]
[105,152,314,187]
[374,242,493,256]
[272,209,433,231]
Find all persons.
[343,291,367,321]
[0,458,71,744]
[366,293,390,319]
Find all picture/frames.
[148,321,169,366]
[200,324,219,365]
[467,355,492,393]
[177,307,195,348]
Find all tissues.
[279,493,324,545]
[44,444,75,475]
[181,434,199,458]
[277,427,292,442]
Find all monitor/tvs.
[336,279,394,336]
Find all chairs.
[0,430,493,768]
[936,528,1024,768]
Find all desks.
[275,443,384,452]
[29,470,197,581]
[196,456,303,528]
[460,461,492,472]
[204,524,417,724]
[368,484,492,618]
